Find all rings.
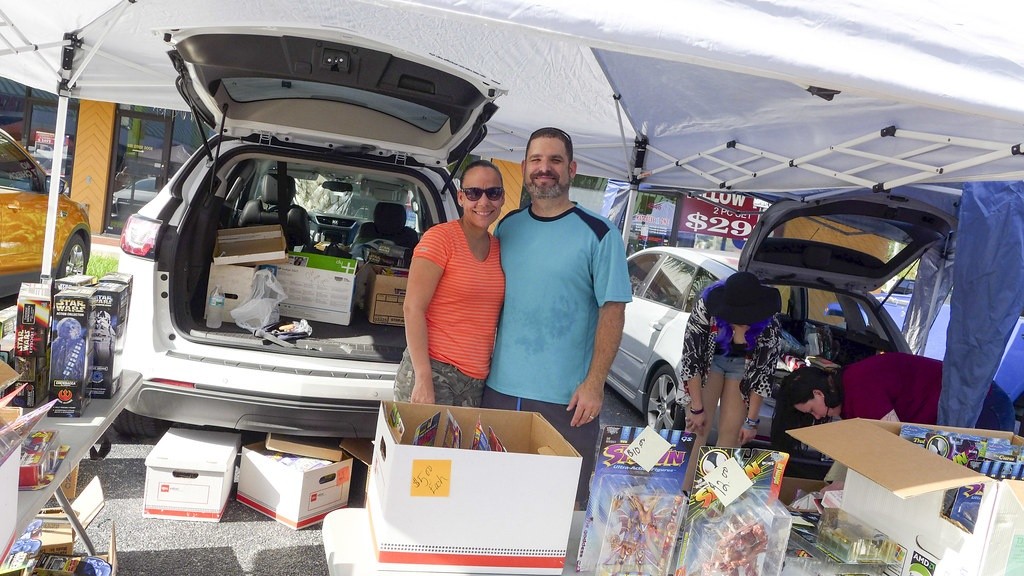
[590,416,594,419]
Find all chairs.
[237,174,310,251]
[353,201,419,268]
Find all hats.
[707,272,780,324]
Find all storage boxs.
[366,266,408,327]
[236,441,354,531]
[366,400,585,575]
[784,417,1024,576]
[0,271,135,576]
[143,428,242,522]
[204,262,258,323]
[212,224,286,267]
[260,251,359,327]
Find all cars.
[605,187,958,442]
[112,177,169,215]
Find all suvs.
[0,129,91,302]
[111,23,508,442]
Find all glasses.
[460,187,503,201]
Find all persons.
[788,353,1016,494]
[395,160,506,408]
[480,128,632,510]
[681,272,781,539]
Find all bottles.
[206,284,225,328]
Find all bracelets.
[743,417,760,430]
[691,407,704,414]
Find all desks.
[14,370,144,557]
[321,507,585,576]
